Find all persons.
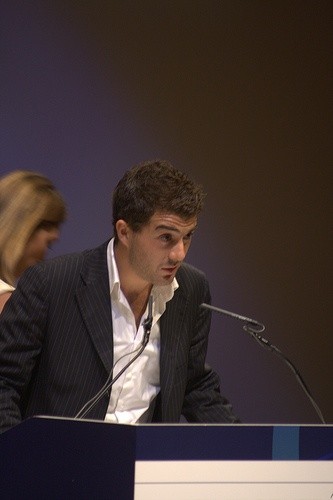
[0,170,66,315]
[0,159,242,433]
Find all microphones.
[200,303,257,326]
[142,295,153,332]
